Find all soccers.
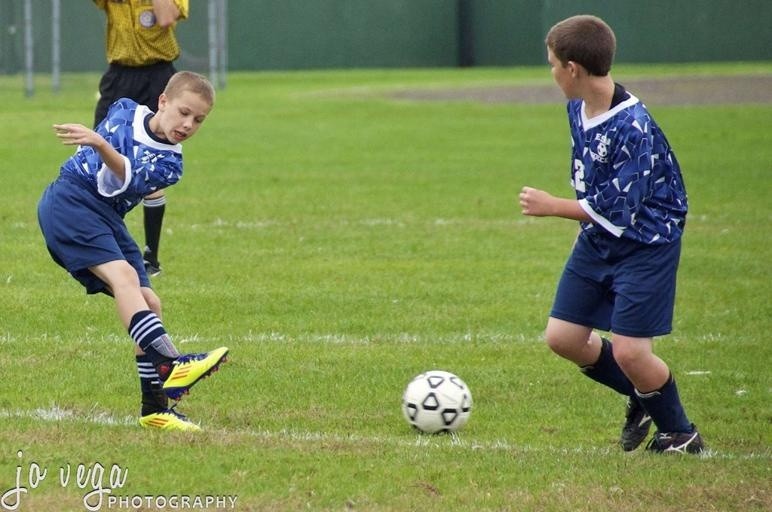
[401,370,471,436]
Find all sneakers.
[652,423,702,453]
[163,346,229,400]
[620,396,653,452]
[140,408,200,432]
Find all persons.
[519,14,704,456]
[91,0,189,280]
[37,71,230,434]
